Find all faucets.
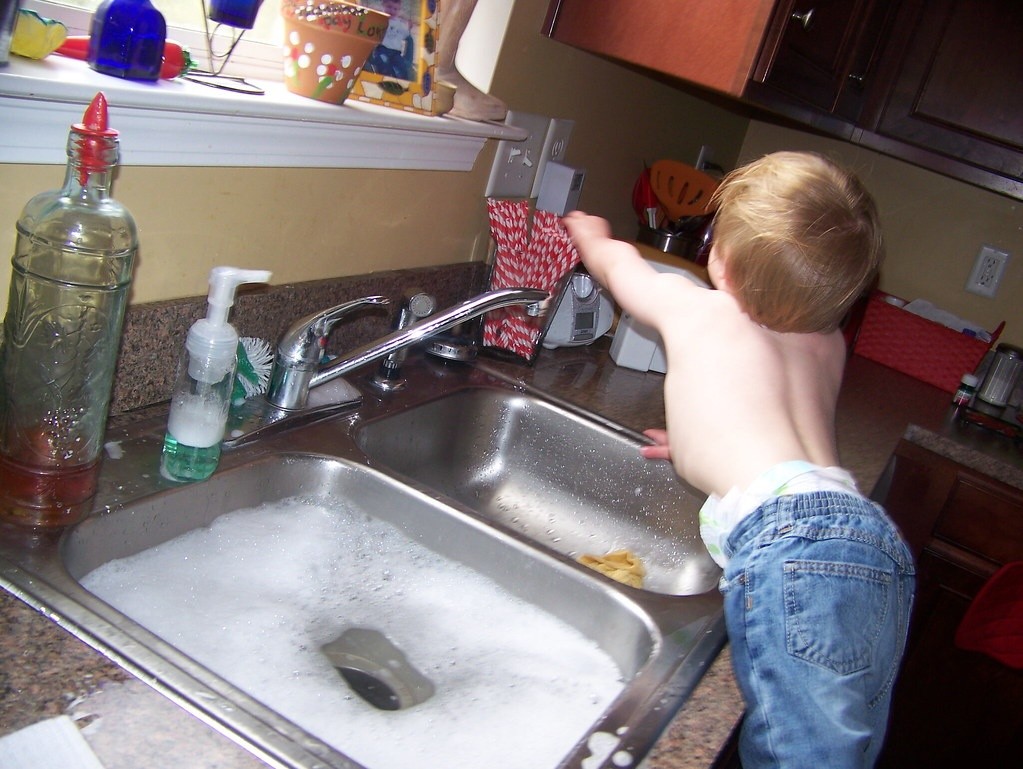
[218,284,551,450]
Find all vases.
[282,0,392,107]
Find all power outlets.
[530,118,576,199]
[964,244,1011,300]
[693,143,716,175]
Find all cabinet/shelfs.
[884,439,1023,769]
[540,0,1023,206]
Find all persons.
[560,149,915,769]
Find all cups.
[635,221,699,263]
[436,79,457,117]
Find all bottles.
[976,342,1023,407]
[0,90,140,529]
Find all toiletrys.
[160,264,273,481]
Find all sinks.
[351,381,723,598]
[52,453,663,768]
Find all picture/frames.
[345,0,441,117]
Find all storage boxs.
[608,310,668,376]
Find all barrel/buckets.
[282,1,391,105]
